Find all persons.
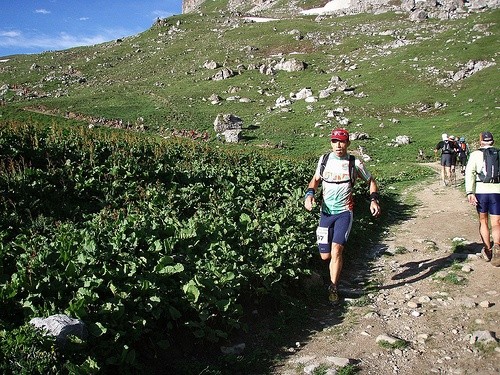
[465,132,500,266]
[435,134,470,186]
[304,128,380,305]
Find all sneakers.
[328,286,341,308]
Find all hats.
[482,132,493,141]
[331,129,349,141]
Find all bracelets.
[305,188,315,199]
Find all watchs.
[371,199,379,204]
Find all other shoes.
[481,246,491,262]
[491,244,500,267]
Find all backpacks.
[475,147,500,183]
[442,140,451,154]
